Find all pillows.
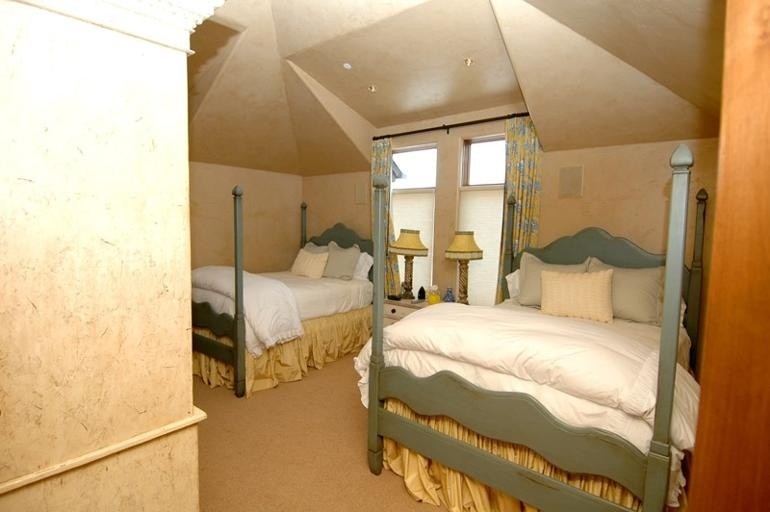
[520,251,591,309]
[589,258,665,325]
[303,242,328,253]
[290,248,328,279]
[539,268,615,323]
[322,241,360,280]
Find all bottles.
[443,288,457,302]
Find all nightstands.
[384,299,444,329]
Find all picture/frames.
[558,166,584,197]
[355,185,368,204]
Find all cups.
[429,286,441,305]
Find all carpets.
[191,358,452,512]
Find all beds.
[367,143,708,512]
[192,185,374,398]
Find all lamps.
[388,229,428,300]
[369,86,377,92]
[445,231,483,306]
[465,58,475,68]
